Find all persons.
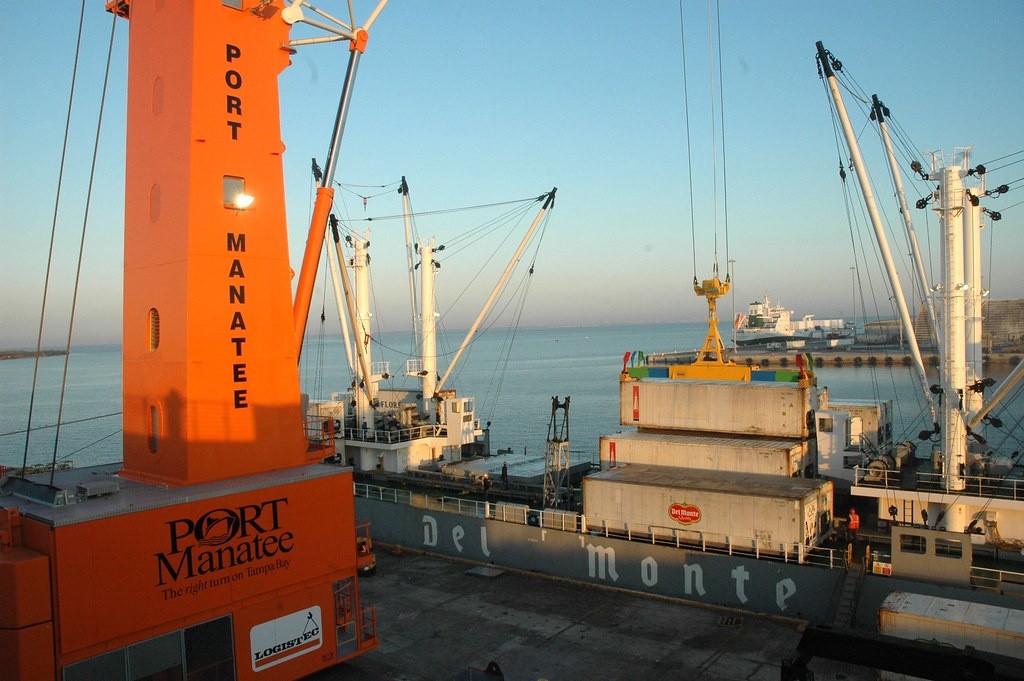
[845,508,860,544]
[483,474,490,500]
[502,462,507,491]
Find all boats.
[293,37,1024,657]
[730,294,849,348]
[823,328,854,348]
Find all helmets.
[850,509,856,515]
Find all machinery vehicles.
[1,0,754,681]
